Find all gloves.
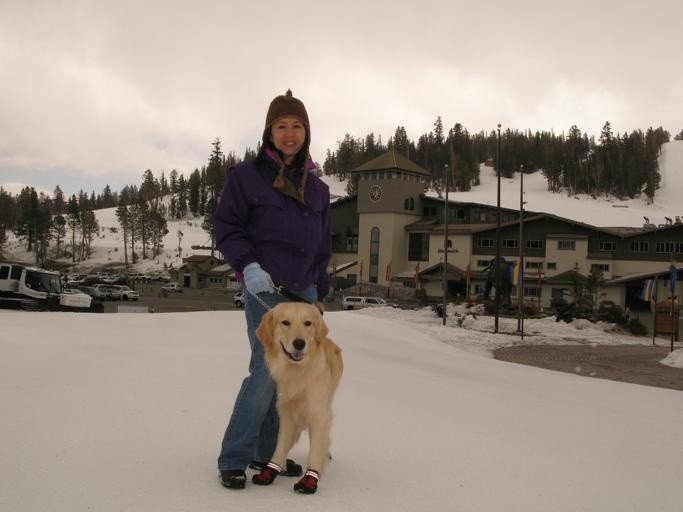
[243,261,276,296]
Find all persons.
[210,96,333,490]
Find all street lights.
[639,250,683,352]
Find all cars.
[0,260,182,313]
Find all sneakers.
[249,456,303,477]
[221,468,247,489]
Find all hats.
[266,95,310,133]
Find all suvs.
[231,290,246,308]
[341,296,400,312]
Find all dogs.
[252,301,344,495]
